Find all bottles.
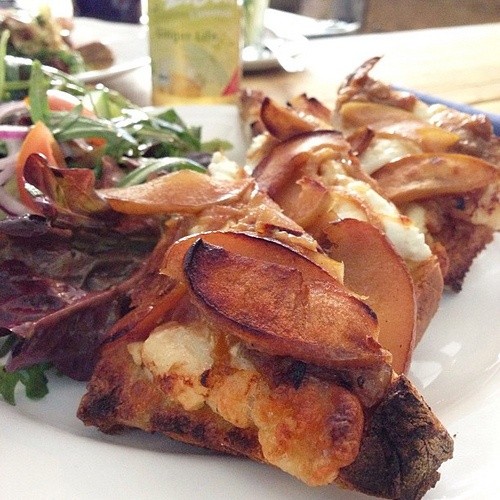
[148,2,248,107]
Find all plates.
[4,210,497,499]
[36,38,148,91]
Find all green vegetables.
[0,30,218,406]
